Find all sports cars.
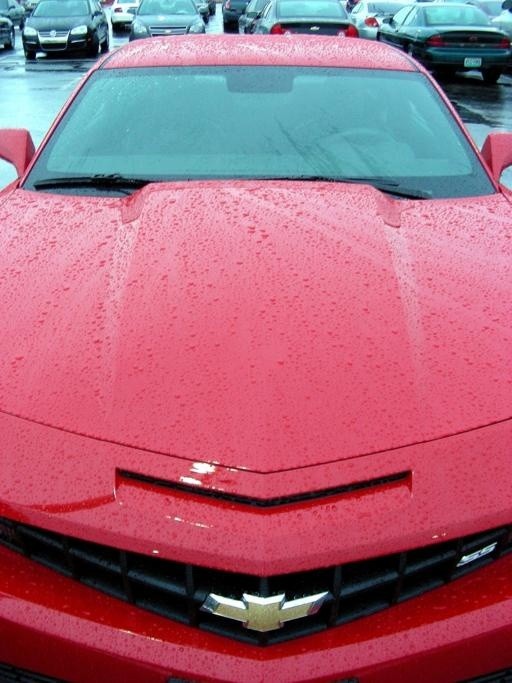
[0,41,512,683]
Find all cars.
[0,16,17,51]
[375,1,512,85]
[21,0,110,60]
